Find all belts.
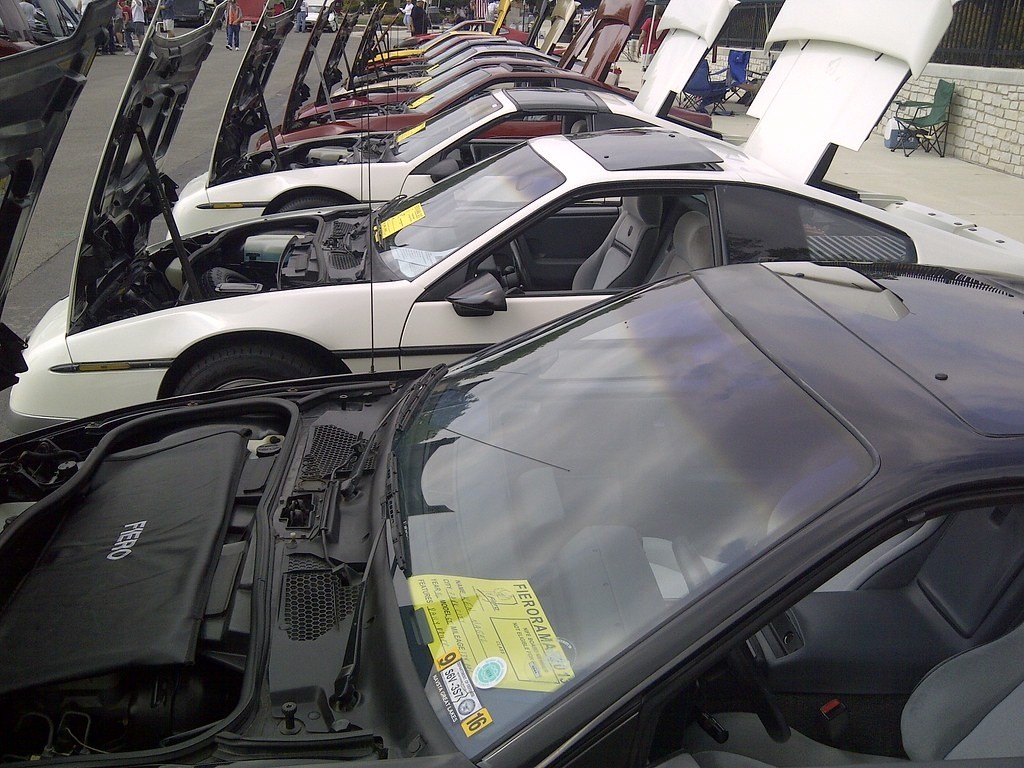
[133,22,144,23]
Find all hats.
[406,0,411,2]
[232,0,236,3]
[417,0,425,3]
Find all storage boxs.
[884,118,919,149]
[240,21,252,32]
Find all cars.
[1,263,1024,768]
[14,27,920,431]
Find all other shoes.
[226,45,232,50]
[102,52,108,55]
[303,30,306,33]
[295,30,299,33]
[236,47,239,51]
[125,49,135,56]
[110,52,115,55]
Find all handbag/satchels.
[125,20,135,32]
[604,63,621,87]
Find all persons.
[101,0,538,56]
[635,5,670,75]
[18,0,37,28]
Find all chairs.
[638,450,1024,767]
[890,79,955,158]
[571,194,714,289]
[677,49,769,115]
